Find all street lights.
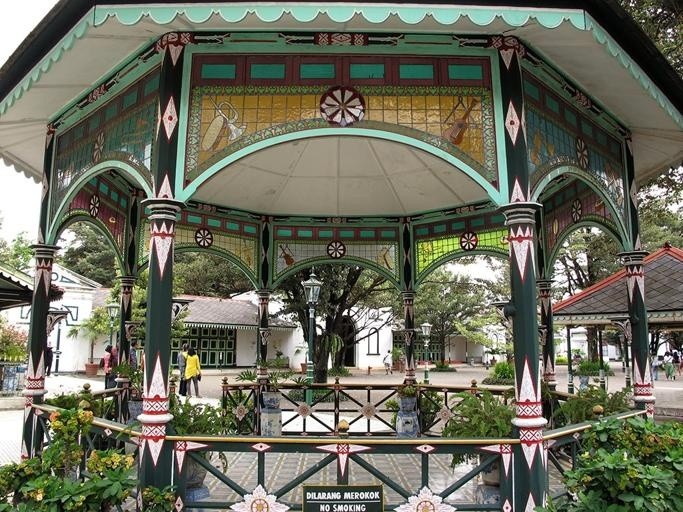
[301,267,323,405]
[419,319,432,398]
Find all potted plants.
[115,393,238,487]
[390,381,422,411]
[439,386,519,485]
[235,356,315,409]
[391,347,409,369]
[294,338,310,374]
[0,313,30,395]
[66,306,121,377]
[107,360,144,398]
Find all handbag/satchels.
[197,374,201,381]
[99,358,105,367]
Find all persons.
[383,349,393,375]
[102,340,139,390]
[45,347,53,376]
[176,344,189,396]
[181,347,204,398]
[652,349,683,382]
[398,352,406,373]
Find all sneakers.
[178,391,203,399]
